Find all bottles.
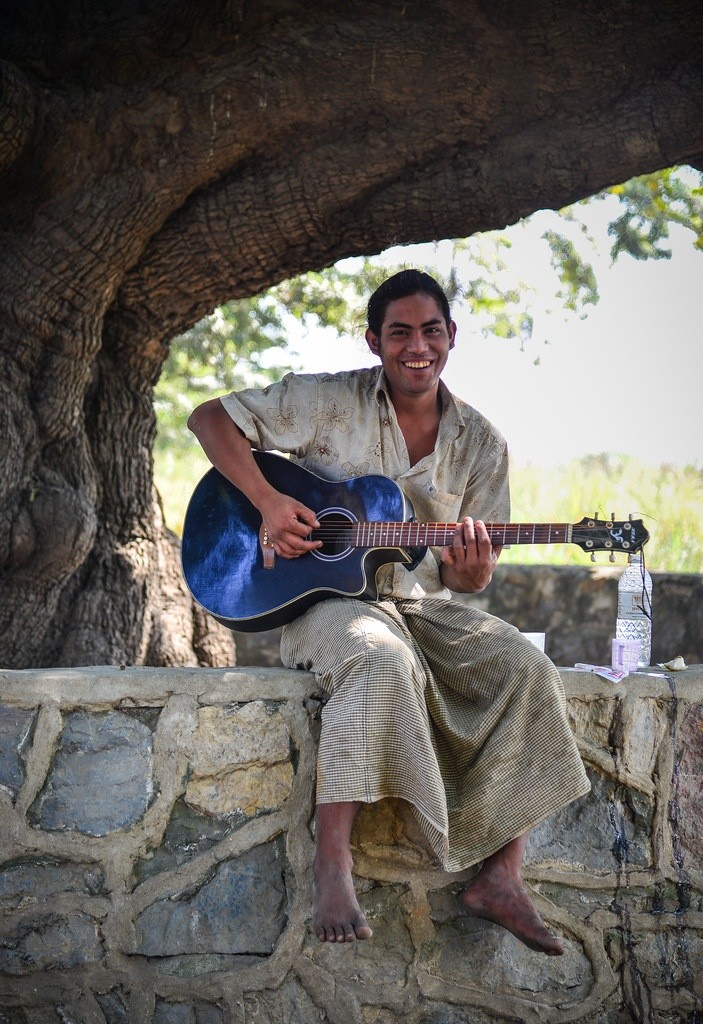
[614,554,653,669]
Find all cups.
[611,638,639,674]
[521,632,545,653]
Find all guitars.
[181,451,655,635]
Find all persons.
[187,270,592,956]
